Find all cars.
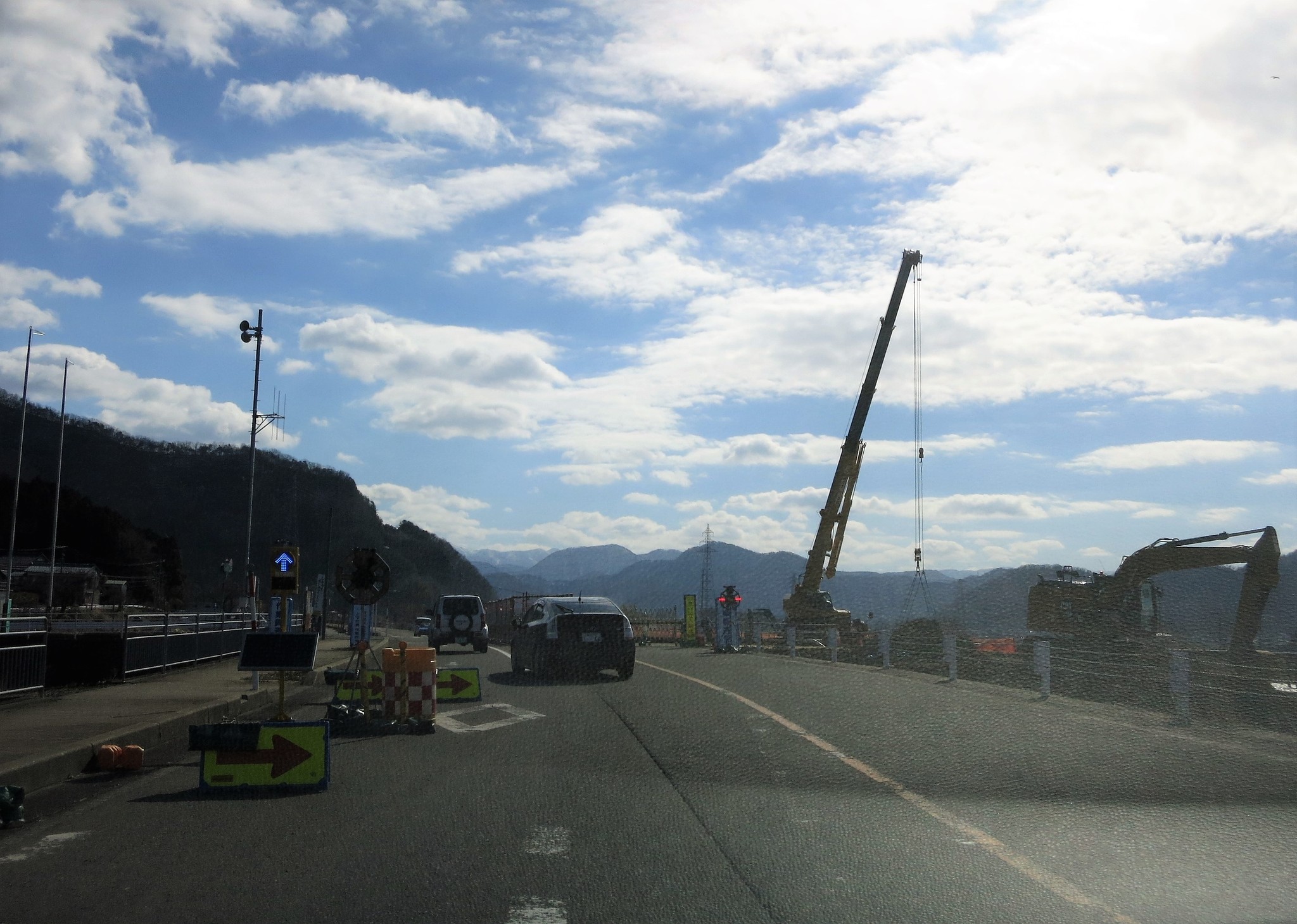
[510,596,635,684]
[414,617,432,637]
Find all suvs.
[428,594,489,655]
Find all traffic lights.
[270,546,299,594]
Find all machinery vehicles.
[783,248,959,679]
[1020,525,1287,718]
